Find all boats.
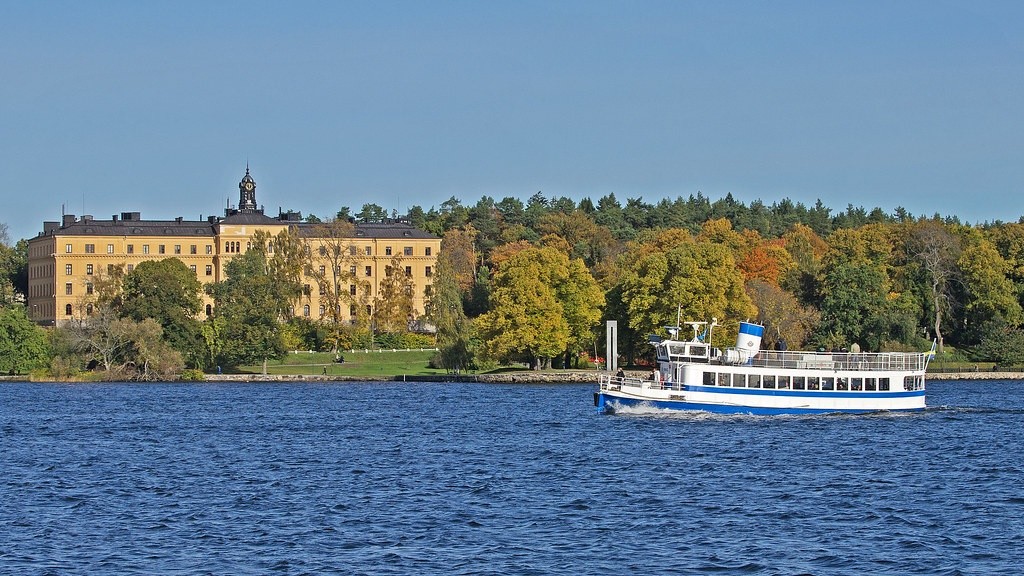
[592,302,936,419]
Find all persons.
[809,382,819,390]
[216,365,222,375]
[321,366,327,375]
[648,371,655,380]
[837,382,843,389]
[774,338,787,360]
[974,364,978,372]
[335,353,344,363]
[754,381,760,388]
[851,383,862,390]
[831,345,849,370]
[850,339,860,371]
[780,381,790,389]
[616,368,625,391]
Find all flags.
[923,340,936,361]
